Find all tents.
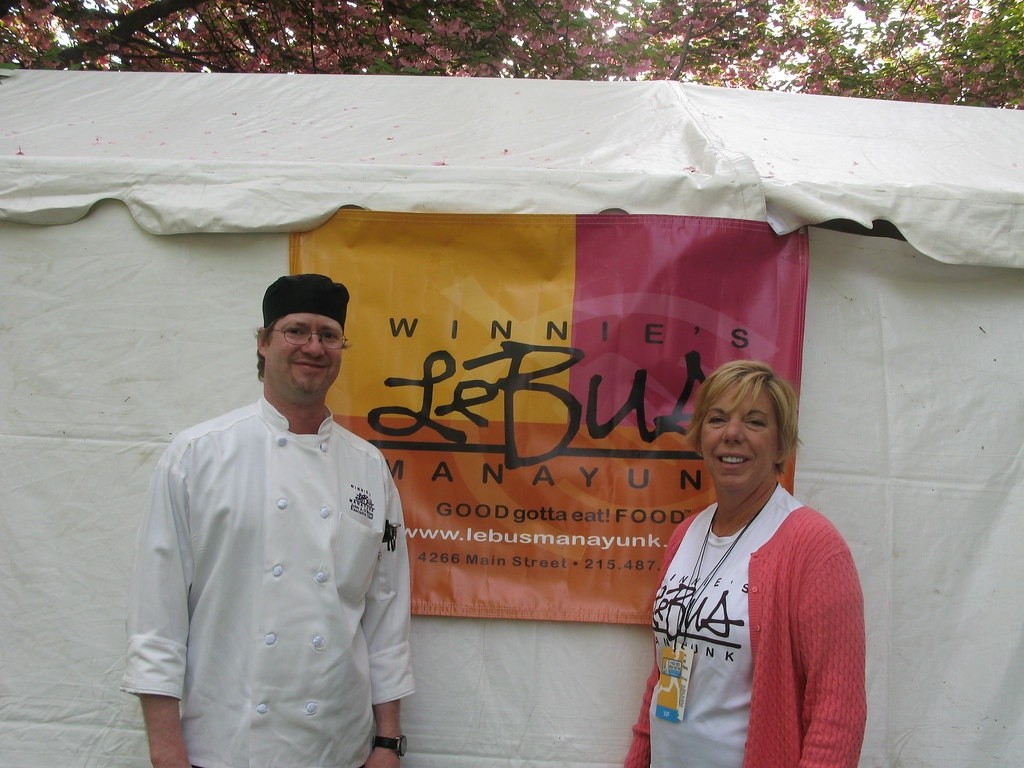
[0,68,1024,768]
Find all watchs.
[372,735,408,758]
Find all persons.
[120,273,415,768]
[624,360,867,768]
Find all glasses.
[271,324,350,350]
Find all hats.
[261,272,350,332]
